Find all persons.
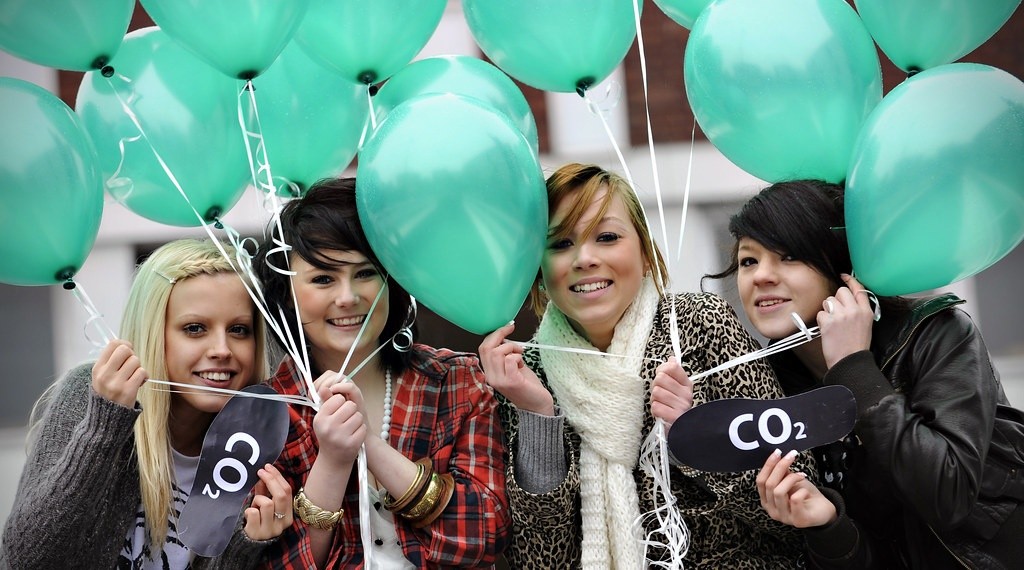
[697,177,1024,570]
[244,177,512,570]
[0,238,294,570]
[478,163,816,570]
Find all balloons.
[0,76,104,291]
[295,0,539,166]
[842,62,1024,297]
[854,0,1022,79]
[74,24,253,228]
[652,0,884,189]
[139,0,369,199]
[461,0,645,97]
[354,94,550,335]
[0,0,136,79]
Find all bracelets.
[292,487,344,532]
[383,455,454,528]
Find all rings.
[274,512,287,519]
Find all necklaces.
[380,368,391,442]
